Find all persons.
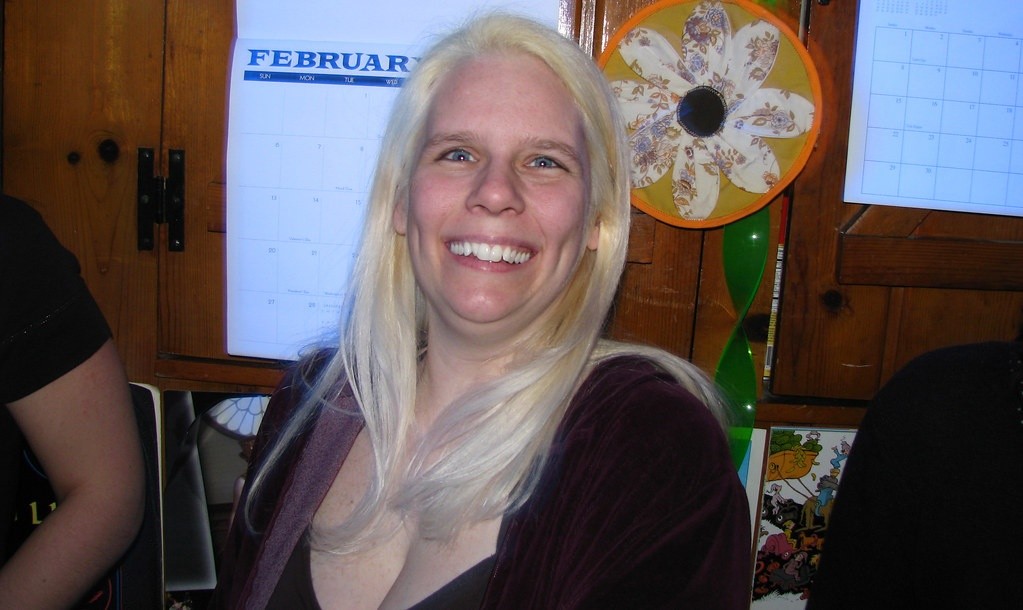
[213,14,755,610]
[0,194,147,610]
[805,336,1023,610]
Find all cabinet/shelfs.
[1,1,1022,603]
